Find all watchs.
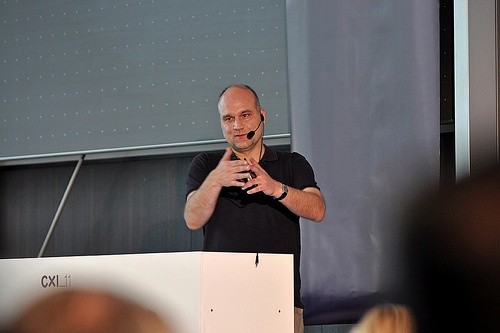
[273,183,288,200]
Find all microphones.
[247,114,264,139]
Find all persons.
[344,160,500,333]
[183,84,325,332]
[9,289,173,333]
